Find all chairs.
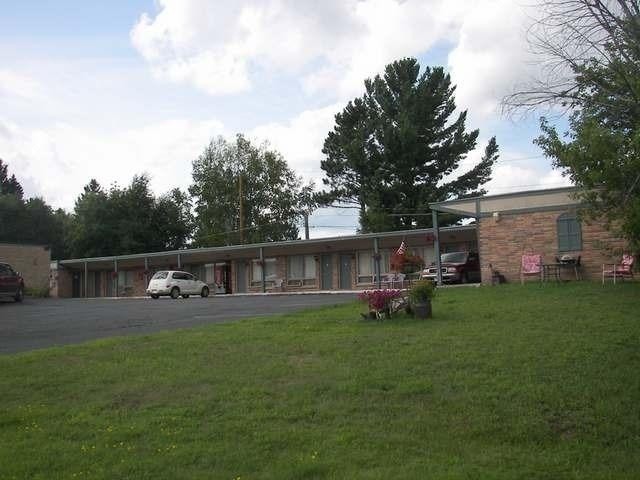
[602,252,635,286]
[555,252,584,283]
[520,254,544,285]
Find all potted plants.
[409,279,434,319]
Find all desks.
[536,262,568,283]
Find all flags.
[393,238,410,258]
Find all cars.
[0,261,26,303]
[145,270,210,299]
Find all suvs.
[421,251,477,286]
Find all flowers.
[357,287,400,309]
